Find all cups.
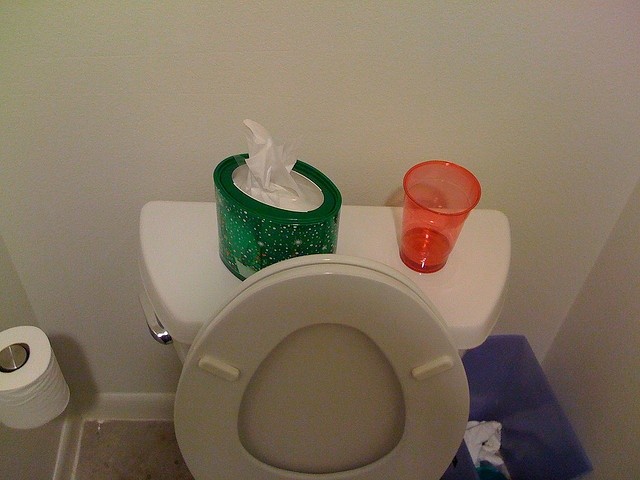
[400,160,482,272]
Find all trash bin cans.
[443,334,593,479]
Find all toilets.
[135,199,510,480]
